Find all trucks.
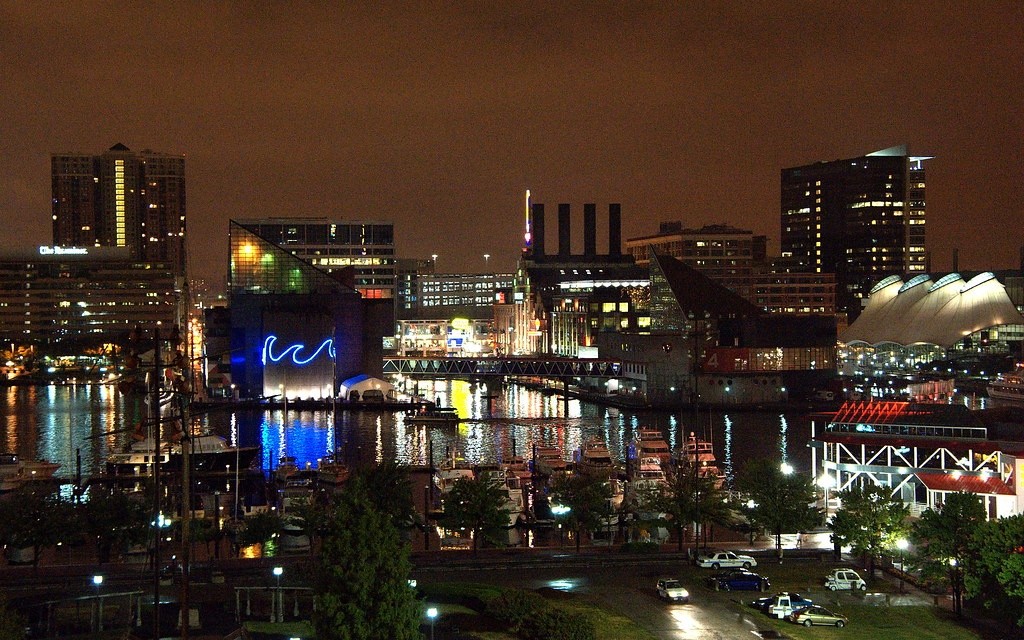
[769,594,820,618]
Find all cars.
[656,577,689,604]
[705,568,771,593]
[697,551,758,570]
[823,569,868,592]
[751,590,813,609]
[791,602,850,629]
